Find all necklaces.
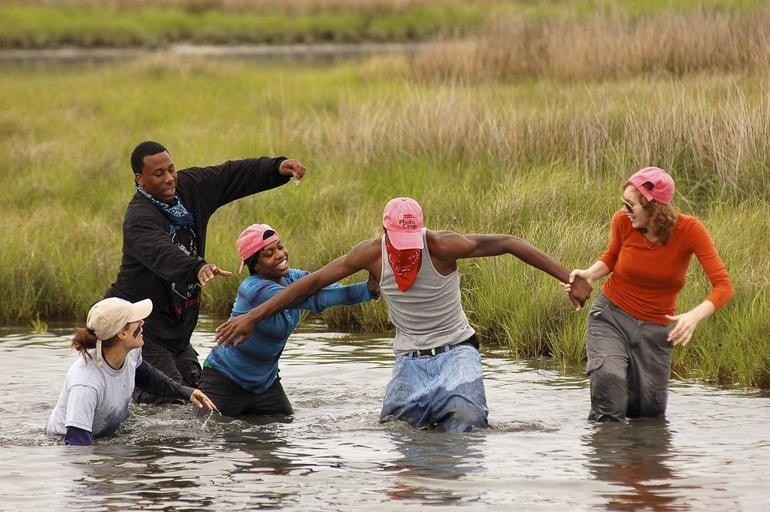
[642,233,663,250]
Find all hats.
[87,297,154,367]
[630,167,675,204]
[237,224,279,273]
[383,198,423,250]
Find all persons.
[192,222,379,419]
[104,140,307,406]
[43,296,221,445]
[214,196,593,435]
[559,166,734,422]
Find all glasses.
[620,196,633,213]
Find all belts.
[405,342,466,357]
